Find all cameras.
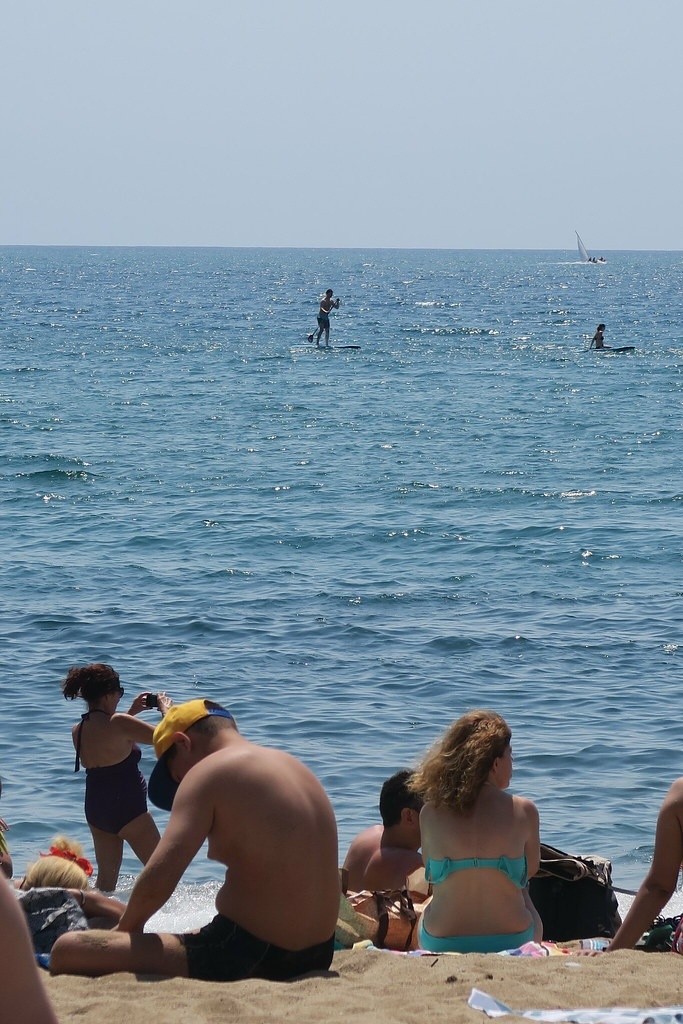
[146,695,158,707]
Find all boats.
[576,346,635,356]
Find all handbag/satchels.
[528,843,622,942]
[347,890,422,952]
[15,888,89,953]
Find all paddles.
[307,298,340,343]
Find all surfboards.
[588,345,636,351]
[293,343,362,349]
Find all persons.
[0,662,683,1024]
[590,324,605,349]
[598,257,605,262]
[315,289,340,349]
[593,257,595,261]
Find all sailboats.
[574,228,608,265]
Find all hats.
[148,700,233,810]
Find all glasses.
[114,687,124,698]
